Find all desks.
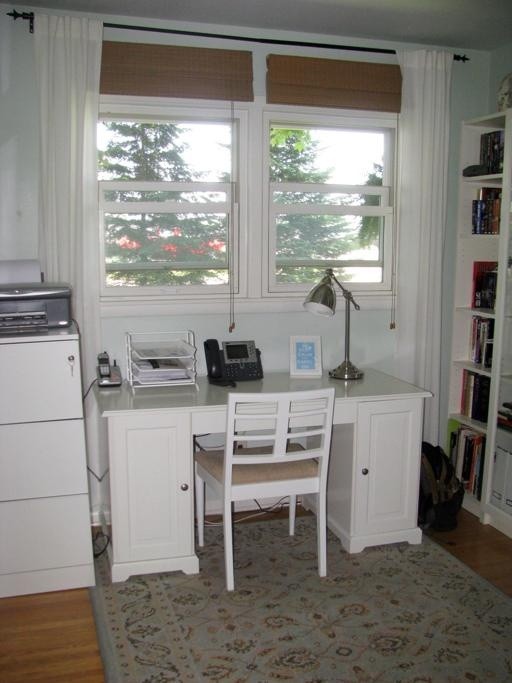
[91,365,433,583]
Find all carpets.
[90,512,511,681]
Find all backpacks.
[418,441,465,532]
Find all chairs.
[193,385,336,593]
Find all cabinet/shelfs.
[444,105,512,540]
[0,318,96,599]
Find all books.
[446,129,505,500]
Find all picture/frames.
[289,334,323,380]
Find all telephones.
[97,350,123,387]
[205,340,264,381]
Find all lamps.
[303,268,365,380]
